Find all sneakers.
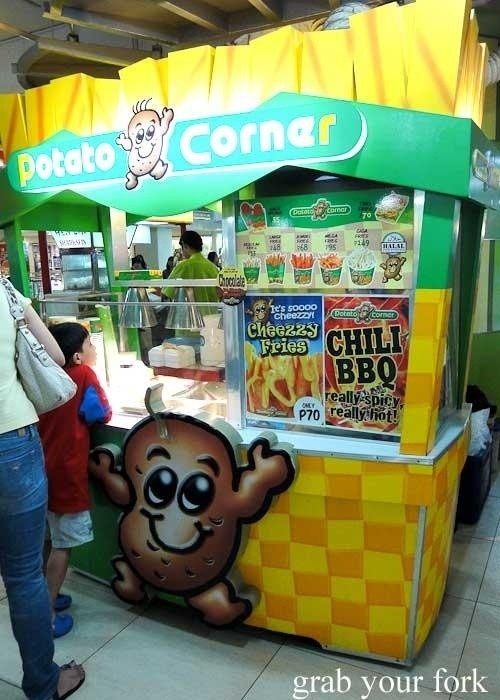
[52,613,74,638]
[55,592,73,612]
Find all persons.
[132,254,149,271]
[30,321,114,641]
[0,240,86,699]
[207,251,222,272]
[161,230,224,344]
[162,256,176,280]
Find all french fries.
[242,257,260,265]
[318,253,342,269]
[291,253,316,269]
[267,253,284,264]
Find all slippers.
[50,659,86,700]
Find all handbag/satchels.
[14,326,78,417]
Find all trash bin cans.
[456,428,494,525]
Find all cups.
[90,316,101,342]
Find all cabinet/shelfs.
[38,301,472,670]
